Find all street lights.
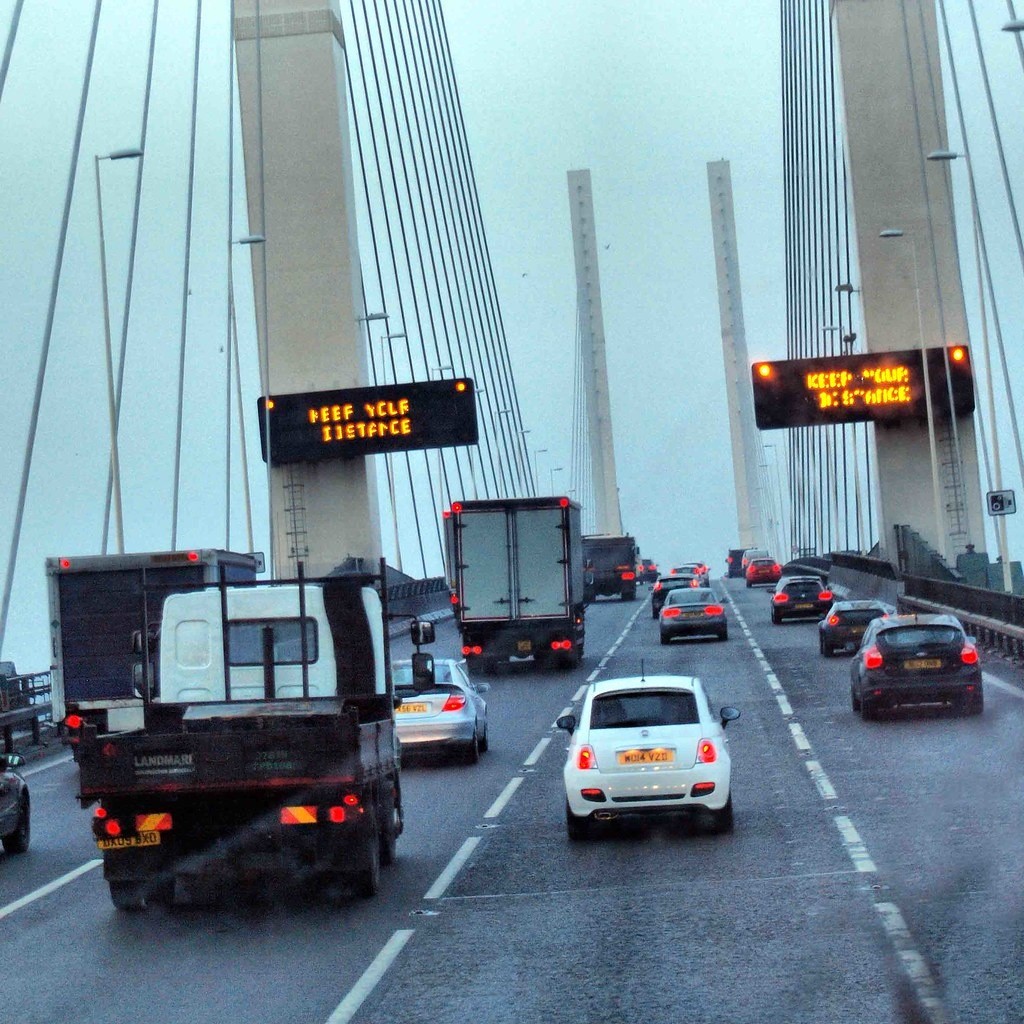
[927,149,1014,596]
[432,365,454,510]
[94,148,144,556]
[764,444,788,563]
[550,467,563,495]
[380,333,409,572]
[880,228,946,565]
[494,408,511,498]
[225,235,267,554]
[533,448,548,496]
[515,429,531,497]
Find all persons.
[659,696,693,721]
[887,631,899,644]
[598,699,627,722]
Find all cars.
[557,674,742,840]
[640,558,660,585]
[765,574,836,625]
[843,611,984,721]
[394,658,492,766]
[656,587,730,644]
[0,741,32,854]
[647,561,712,620]
[744,556,785,585]
[817,599,891,659]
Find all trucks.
[72,553,437,915]
[581,532,639,602]
[42,550,257,766]
[441,495,595,676]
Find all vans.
[725,547,757,579]
[738,550,769,579]
